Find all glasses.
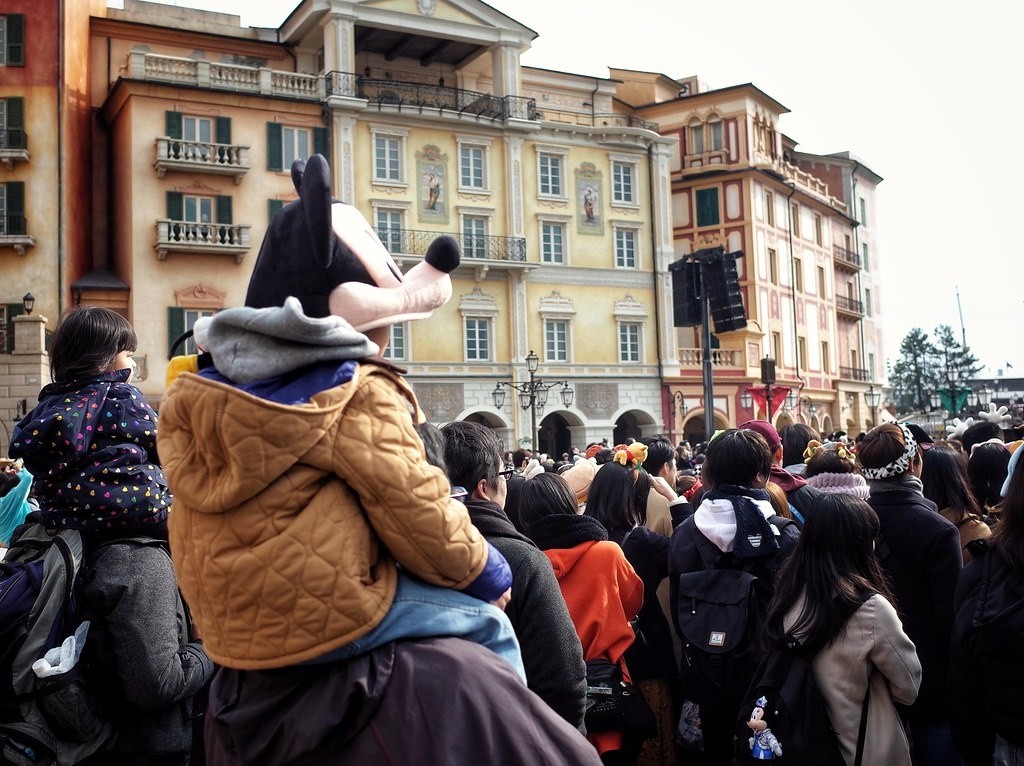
[475,465,514,488]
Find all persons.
[153,153,529,682]
[0,421,1024,766]
[7,306,172,549]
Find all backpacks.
[676,513,800,705]
[0,518,119,766]
[730,586,881,766]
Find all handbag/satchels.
[634,679,678,766]
[583,658,623,730]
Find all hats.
[738,420,782,454]
[562,460,598,498]
[243,153,460,331]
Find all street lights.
[865,384,881,427]
[1008,395,1015,427]
[491,350,574,458]
[942,409,951,438]
[761,355,776,425]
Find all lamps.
[23,293,35,314]
[13,398,27,426]
